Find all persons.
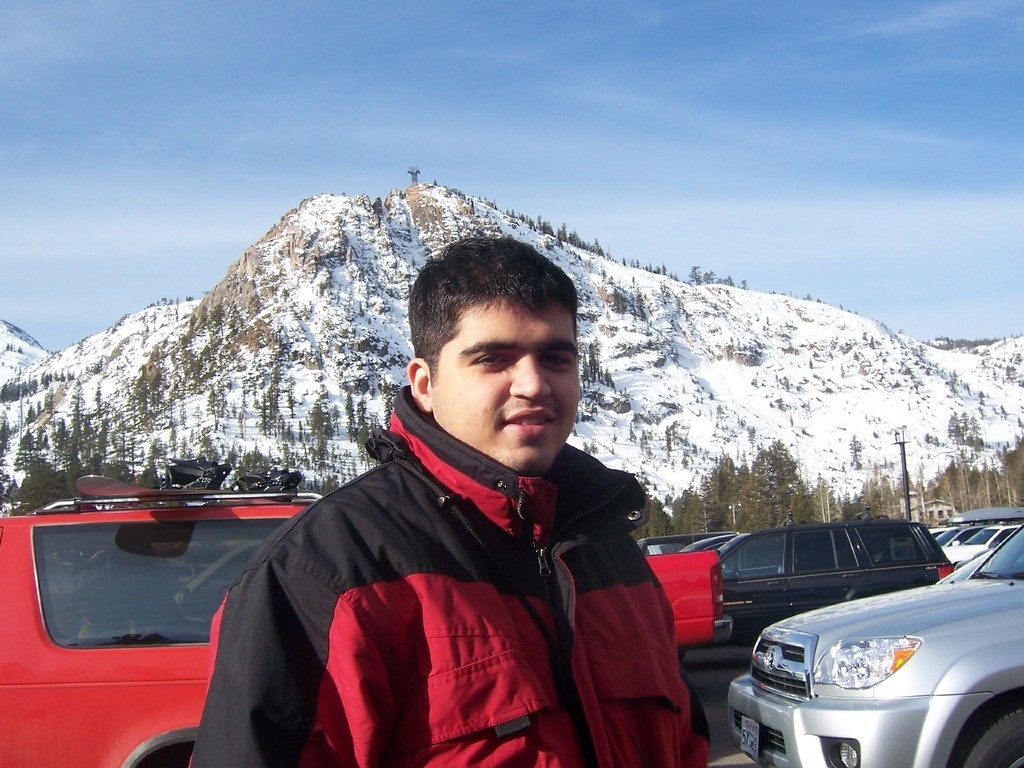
[180,236,710,768]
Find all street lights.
[893,425,910,520]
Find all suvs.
[0,455,735,768]
[726,521,1024,768]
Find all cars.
[634,500,1024,648]
